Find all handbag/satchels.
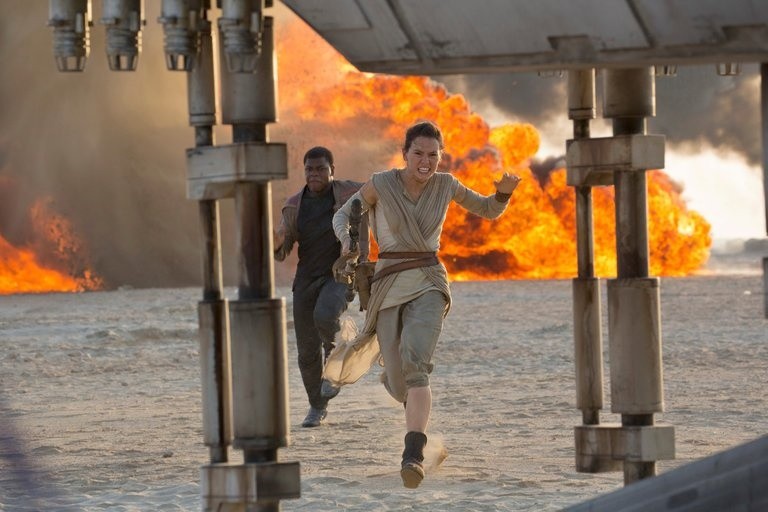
[354,261,377,310]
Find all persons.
[270,147,372,427]
[333,121,523,489]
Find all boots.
[400,431,428,489]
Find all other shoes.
[302,409,328,426]
[321,380,340,398]
